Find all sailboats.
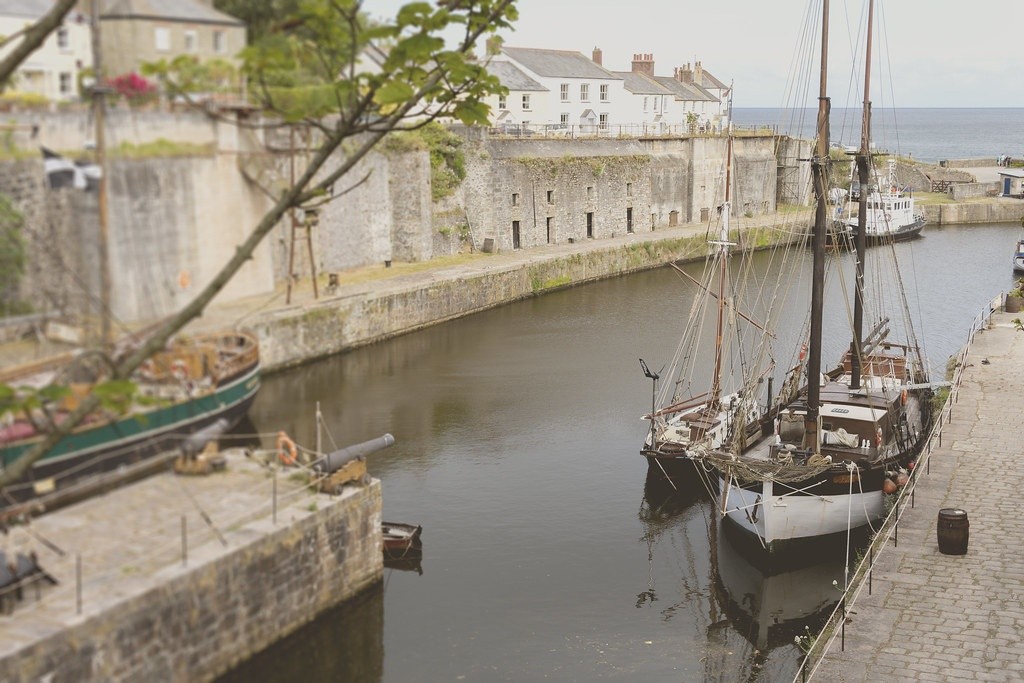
[705,0,942,578]
[705,499,885,683]
[627,129,762,495]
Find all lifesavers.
[874,427,883,446]
[274,431,297,465]
[170,359,191,381]
[139,358,155,379]
[799,344,809,363]
[902,389,908,407]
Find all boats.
[1,333,263,506]
[382,519,424,557]
[807,136,927,247]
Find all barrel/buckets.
[937,508,970,555]
